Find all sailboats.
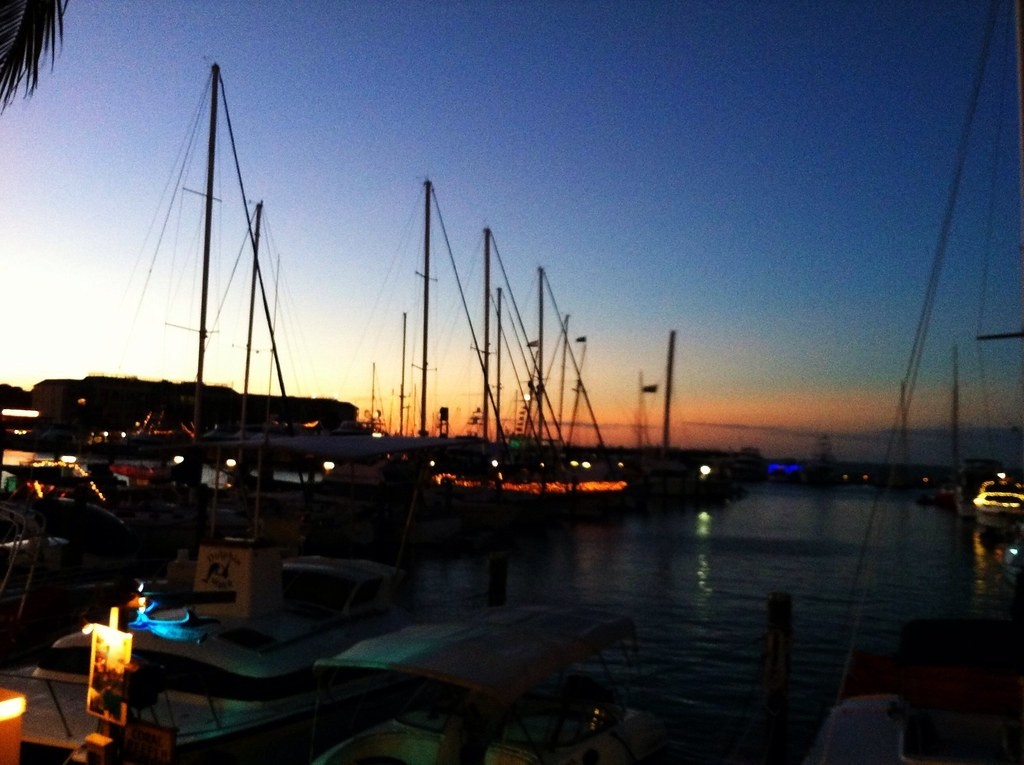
[0,52,1023,764]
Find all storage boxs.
[894,617,1024,720]
